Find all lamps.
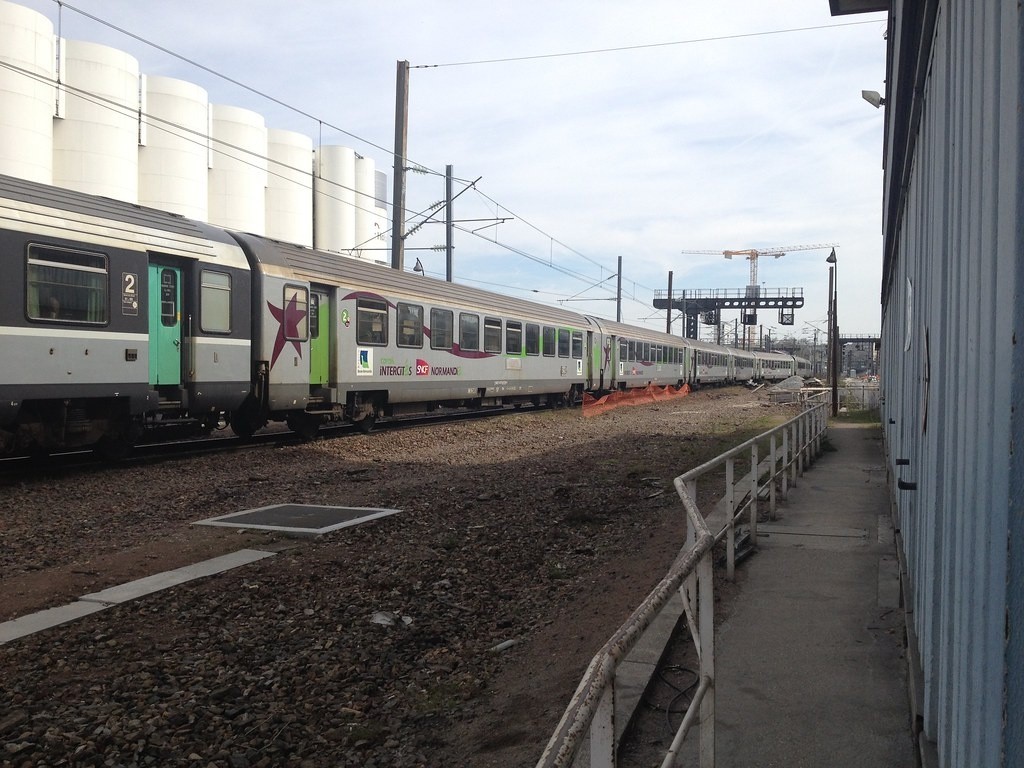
[862,90,885,109]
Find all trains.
[1,173,818,467]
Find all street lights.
[413,258,425,275]
[824,246,838,419]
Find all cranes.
[680,242,840,286]
